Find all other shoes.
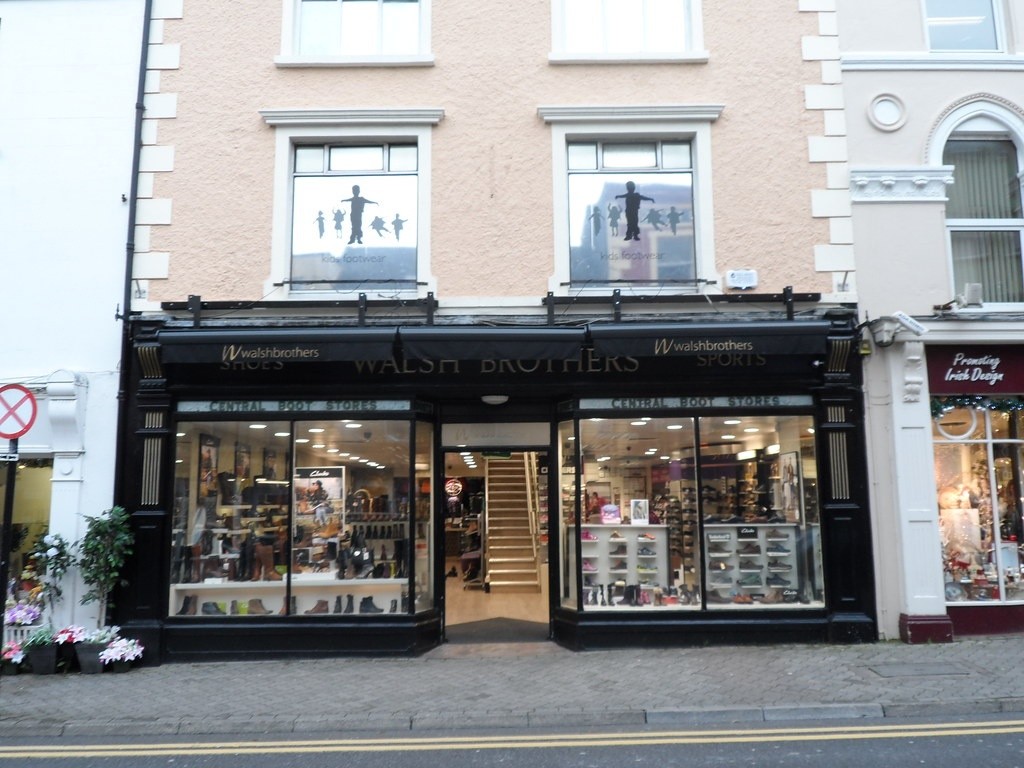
[465,522,477,534]
[720,516,743,523]
[703,515,718,523]
[767,517,787,523]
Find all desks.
[169,577,417,617]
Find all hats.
[312,480,322,487]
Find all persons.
[204,449,212,485]
[783,457,797,486]
[585,489,606,514]
[305,480,334,526]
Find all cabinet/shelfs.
[459,530,482,589]
[737,478,756,508]
[350,521,410,561]
[198,502,278,560]
[567,524,670,606]
[695,522,799,598]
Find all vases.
[113,659,131,673]
[74,642,106,674]
[3,659,20,675]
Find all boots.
[581,583,699,606]
[463,562,479,582]
[344,497,408,521]
[236,542,283,581]
[194,471,289,553]
[176,595,399,615]
[169,543,204,582]
[353,524,404,540]
[337,539,410,580]
[204,556,230,577]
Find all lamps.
[933,282,984,310]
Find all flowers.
[98,636,144,664]
[0,566,44,628]
[28,532,77,605]
[53,624,120,644]
[1,640,25,664]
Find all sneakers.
[581,531,659,589]
[709,529,810,604]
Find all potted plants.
[20,627,58,674]
